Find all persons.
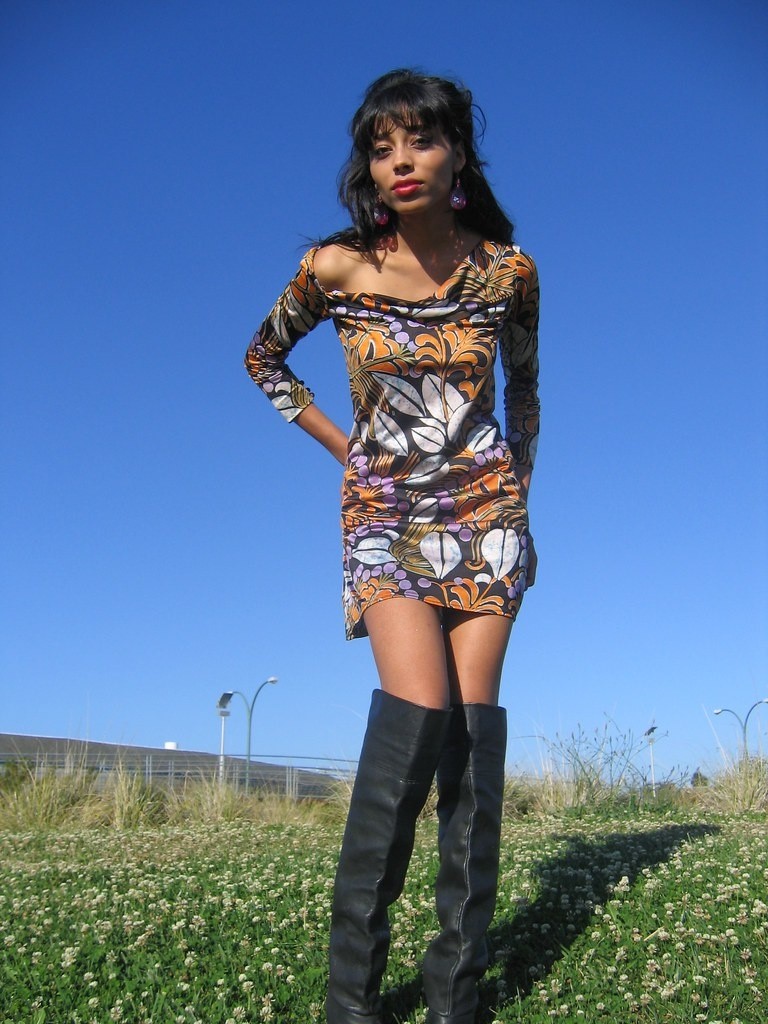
[245,67,542,1023]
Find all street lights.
[713,699,768,762]
[221,677,277,796]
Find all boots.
[328,688,507,1018]
